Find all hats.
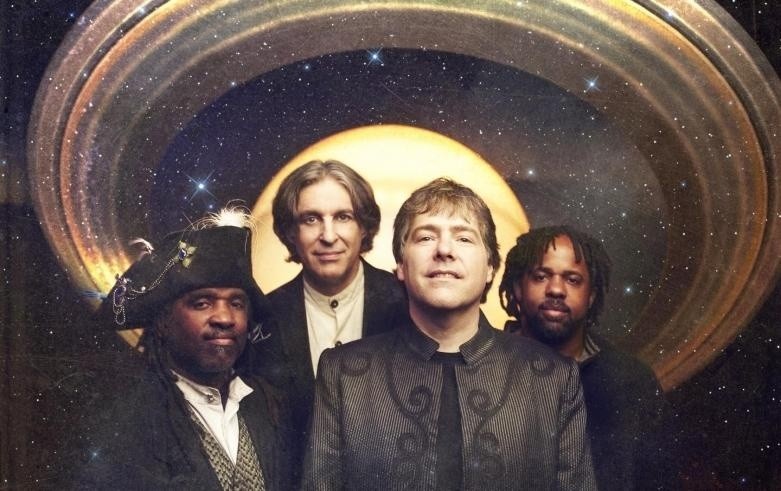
[91,225,253,331]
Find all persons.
[301,178,599,491]
[35,198,305,491]
[499,227,725,491]
[234,159,408,453]
